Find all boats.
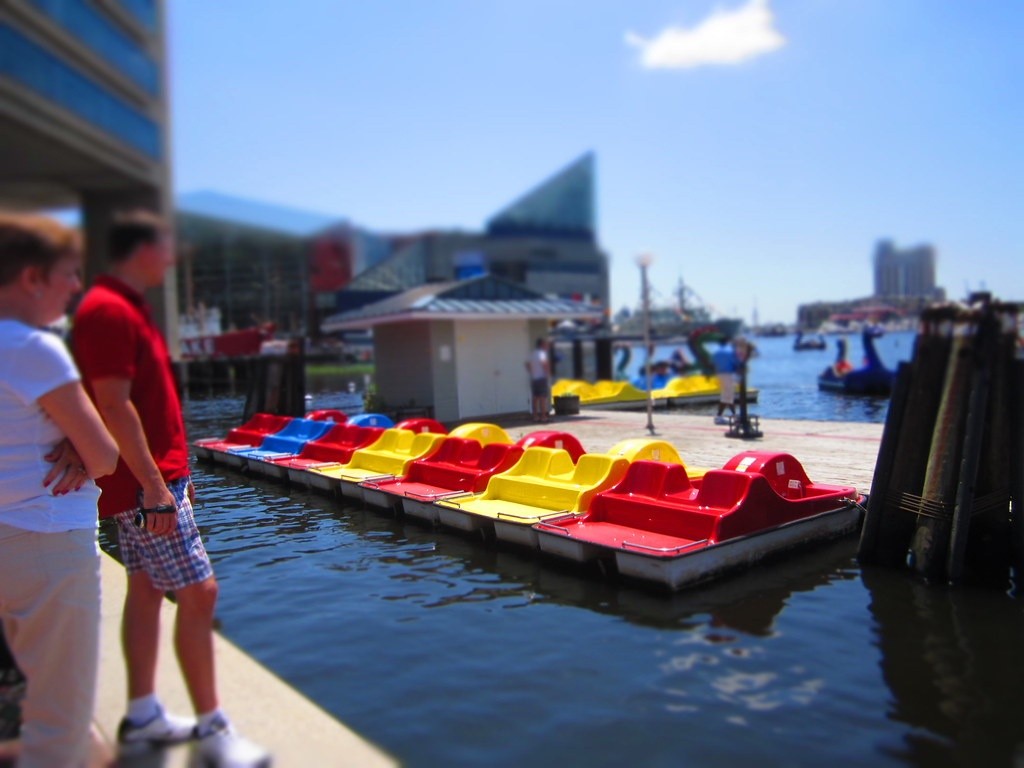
[815,321,895,394]
[307,416,511,502]
[359,415,588,524]
[547,375,666,412]
[192,408,392,480]
[791,328,826,352]
[535,450,866,591]
[433,433,711,545]
[653,375,760,404]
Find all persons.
[0,211,121,768]
[70,209,273,768]
[712,335,741,424]
[525,335,554,423]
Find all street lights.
[637,248,656,430]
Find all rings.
[79,467,86,474]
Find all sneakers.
[188,723,272,768]
[117,705,196,747]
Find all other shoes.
[533,417,554,423]
[714,416,731,425]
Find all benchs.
[548,375,759,404]
[192,412,867,561]
